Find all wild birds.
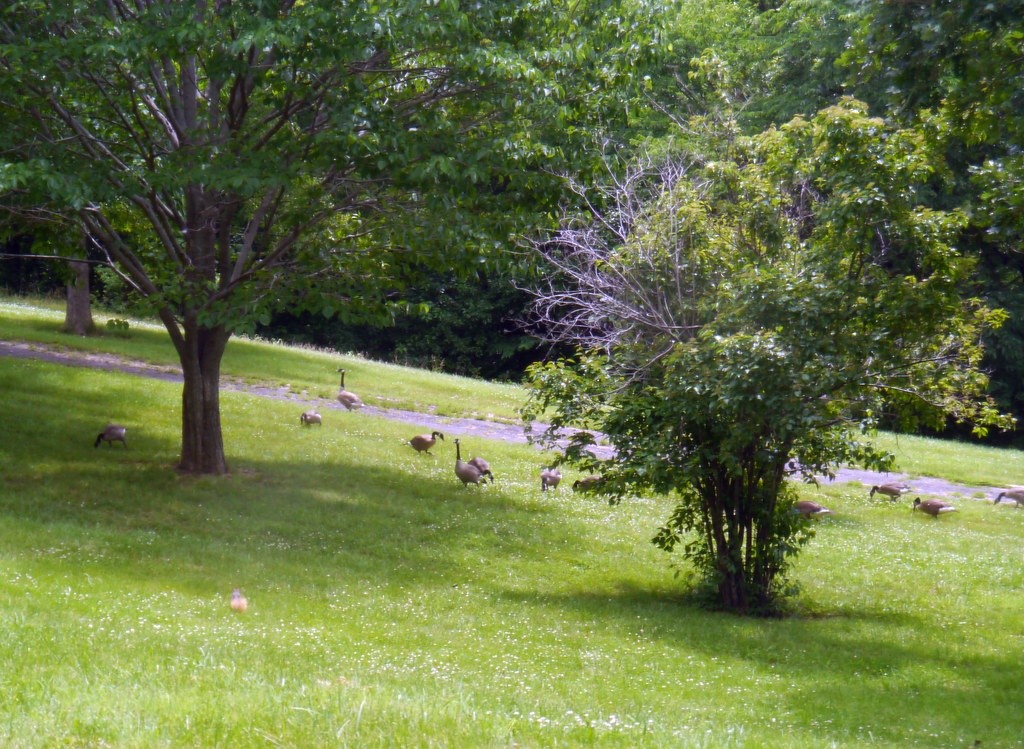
[541,468,562,490]
[914,497,961,518]
[403,431,445,455]
[453,439,494,486]
[335,368,367,413]
[793,501,834,517]
[993,489,1024,508]
[300,410,322,425]
[572,476,602,491]
[870,482,914,503]
[94,425,127,451]
